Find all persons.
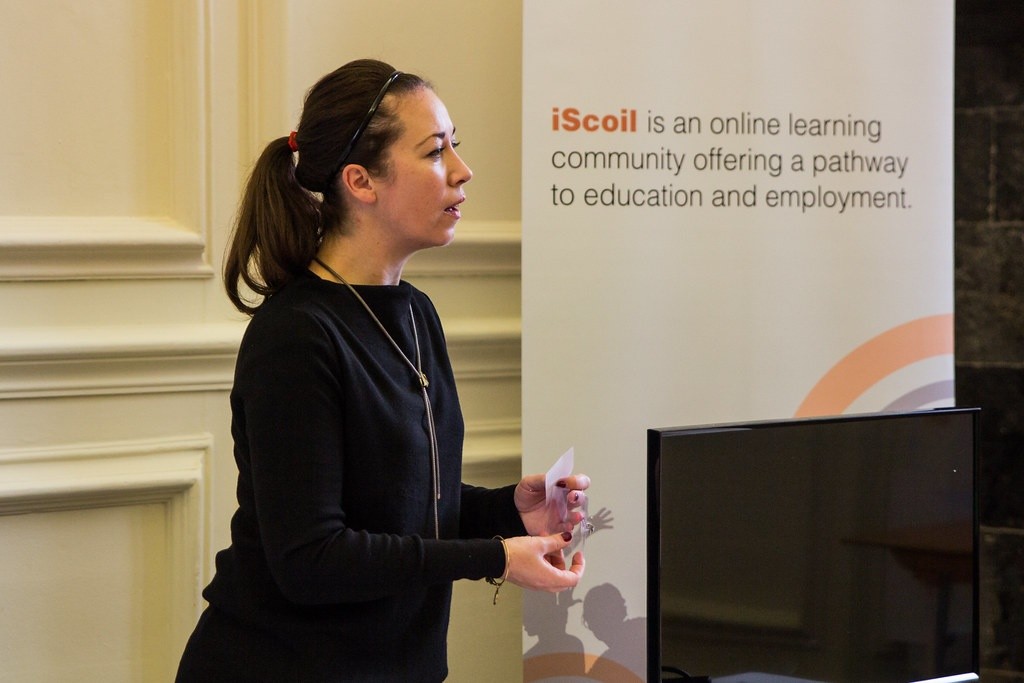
[174,59,588,683]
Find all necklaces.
[319,255,441,541]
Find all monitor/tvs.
[647,405,984,683]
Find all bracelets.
[487,534,510,605]
